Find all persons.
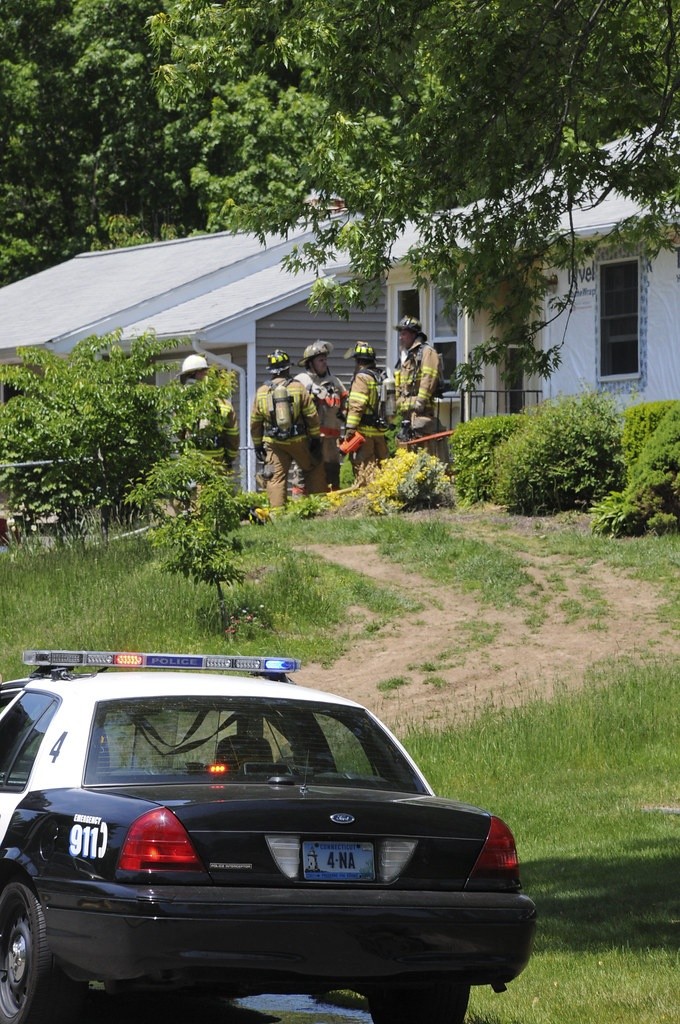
[343,340,391,475]
[172,354,241,497]
[251,349,327,510]
[394,315,440,455]
[292,342,348,501]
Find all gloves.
[310,437,320,451]
[345,429,356,442]
[414,401,423,416]
[255,446,267,460]
[225,459,233,468]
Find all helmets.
[266,349,294,370]
[354,341,375,359]
[297,343,330,368]
[176,354,210,377]
[396,315,428,342]
[249,506,266,526]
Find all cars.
[0,650,539,1024]
